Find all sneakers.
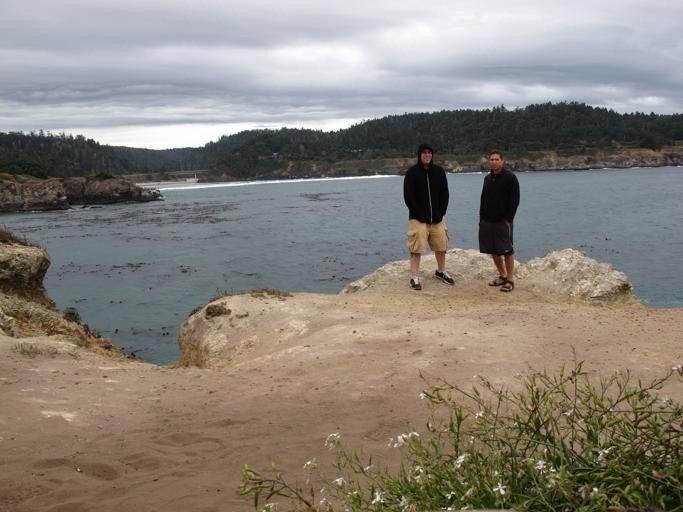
[409,276,421,290]
[435,270,454,285]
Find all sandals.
[489,276,507,286]
[501,280,514,292]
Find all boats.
[185,172,199,183]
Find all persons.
[404,144,455,290]
[479,150,520,292]
